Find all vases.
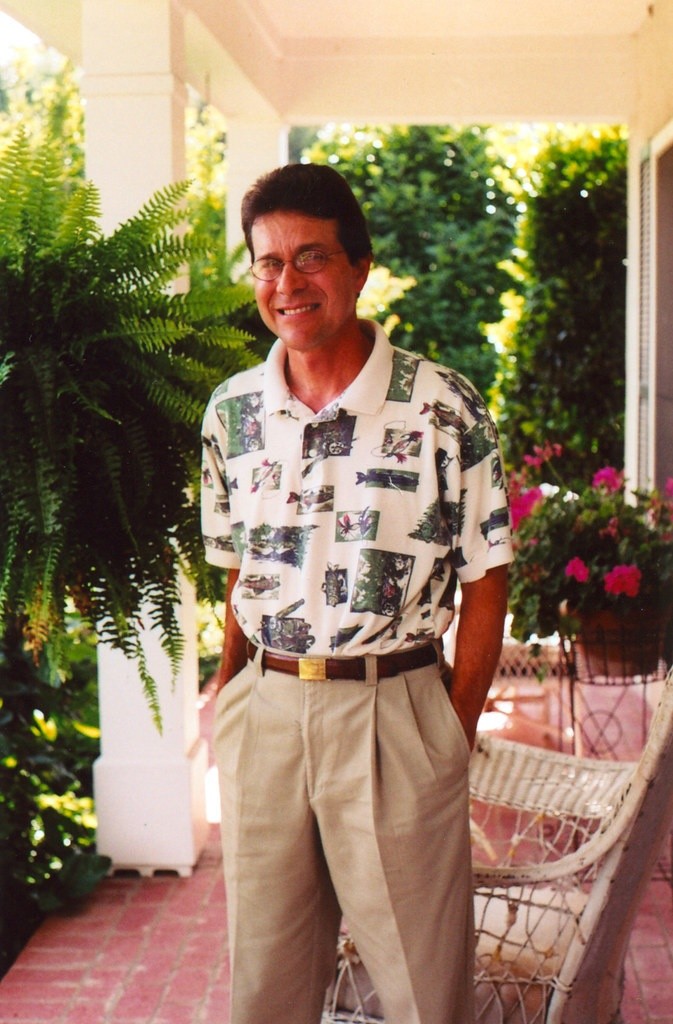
[561,603,665,680]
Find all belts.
[247,642,437,681]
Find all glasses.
[248,248,348,282]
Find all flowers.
[502,439,673,643]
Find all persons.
[216,163,512,1023]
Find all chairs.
[322,665,673,1024]
